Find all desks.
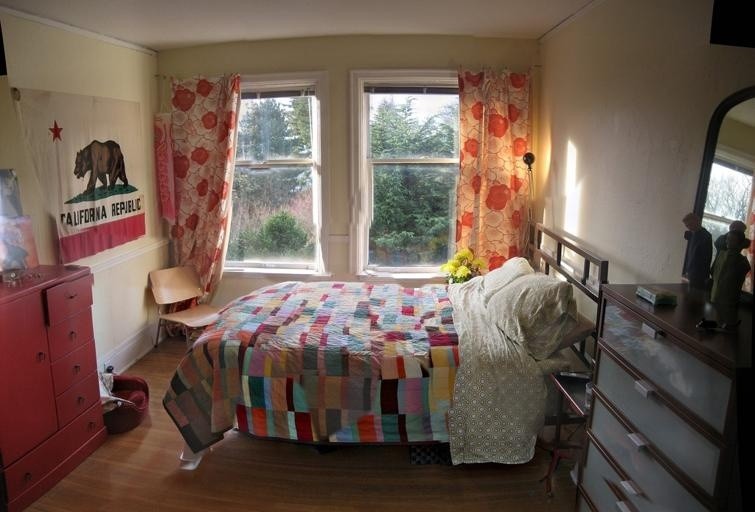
[539,371,593,498]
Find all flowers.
[439,247,484,284]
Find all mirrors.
[681,85,755,311]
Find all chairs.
[149,265,223,348]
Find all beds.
[194,219,609,455]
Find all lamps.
[523,153,535,221]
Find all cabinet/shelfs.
[575,283,754,511]
[0,265,108,512]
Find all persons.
[710,230,751,331]
[710,221,751,277]
[682,213,713,306]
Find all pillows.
[478,256,578,360]
